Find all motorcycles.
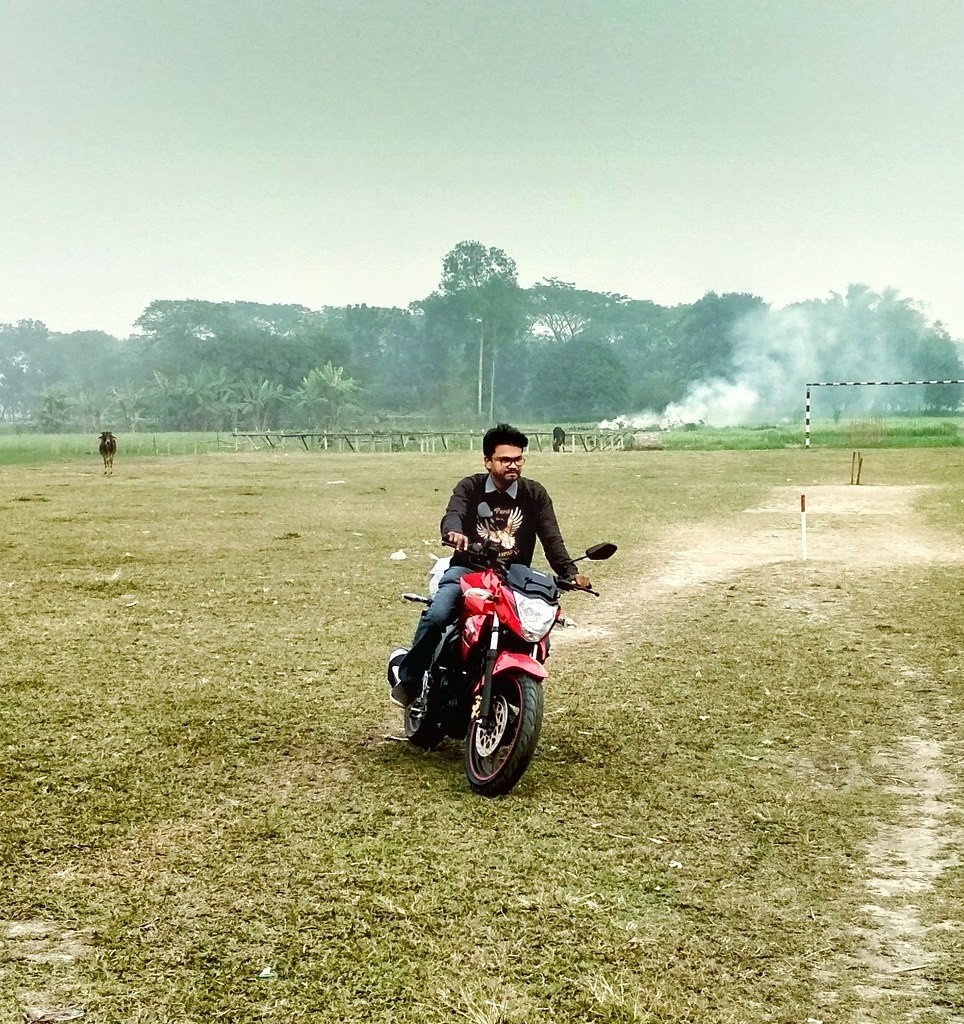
[386,501,619,799]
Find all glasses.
[493,454,526,467]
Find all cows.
[97,431,117,476]
[552,426,565,453]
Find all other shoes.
[389,680,416,709]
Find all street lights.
[470,318,483,415]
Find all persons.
[390,422,592,709]
